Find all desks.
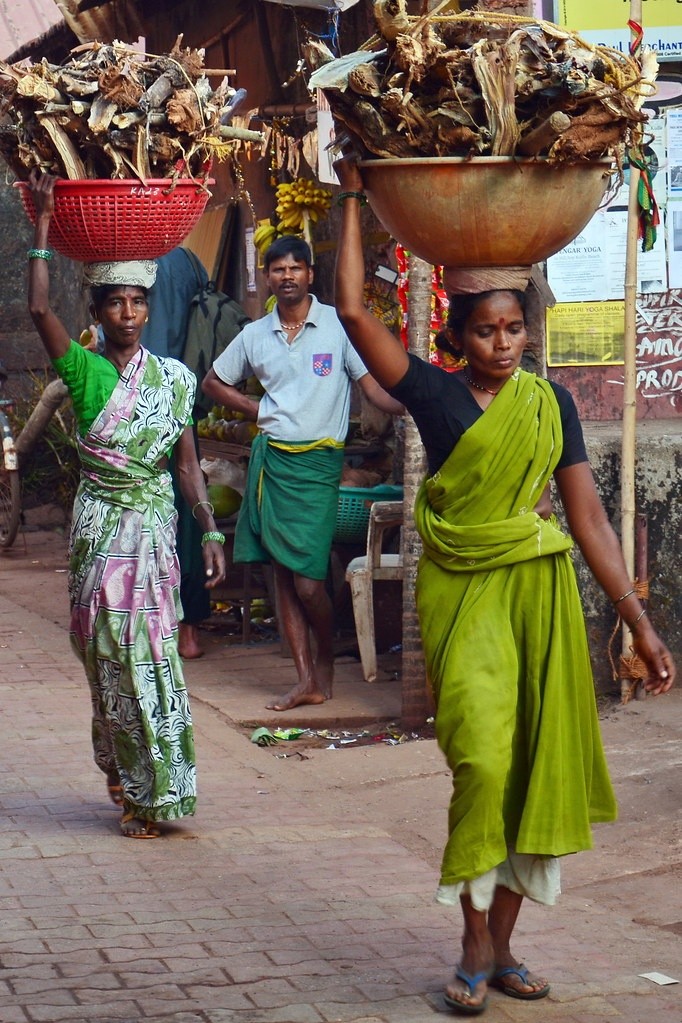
[194,435,387,646]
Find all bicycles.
[1,366,28,557]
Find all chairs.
[345,502,407,684]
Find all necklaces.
[463,365,499,395]
[281,320,305,330]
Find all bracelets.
[200,532,226,549]
[192,501,214,518]
[629,609,648,634]
[334,190,366,208]
[27,248,52,262]
[614,590,635,608]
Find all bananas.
[254,175,336,314]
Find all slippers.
[106,776,125,807]
[442,960,491,1012]
[489,963,550,1000]
[120,810,161,840]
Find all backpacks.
[179,244,254,423]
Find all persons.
[331,158,674,1015]
[27,172,231,838]
[201,232,409,712]
[83,242,215,653]
